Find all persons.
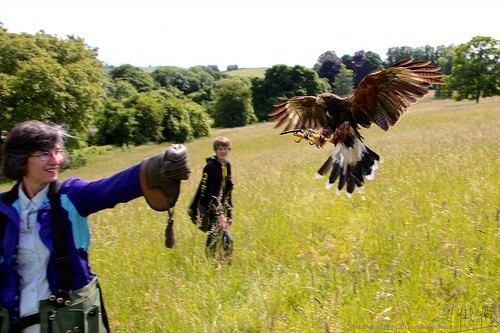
[189,137,234,266]
[0,120,191,333]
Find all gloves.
[146,144,191,188]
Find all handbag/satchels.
[38,275,110,333]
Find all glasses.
[29,150,65,160]
